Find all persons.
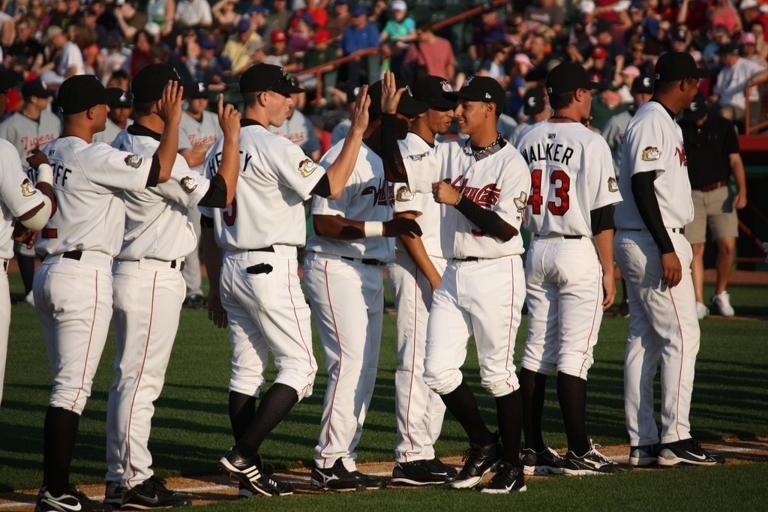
[387,75,459,485]
[615,50,726,467]
[1,80,62,306]
[34,75,183,512]
[677,100,747,321]
[516,64,623,475]
[0,65,56,494]
[106,64,241,511]
[332,83,397,316]
[198,64,370,497]
[602,76,655,319]
[381,70,531,494]
[270,74,323,307]
[302,81,421,492]
[1,1,768,149]
[178,82,221,310]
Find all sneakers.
[219,446,279,497]
[482,465,526,493]
[563,446,634,475]
[311,461,365,492]
[103,481,128,505]
[349,470,386,489]
[713,291,733,316]
[421,459,457,477]
[658,439,725,466]
[391,463,446,484]
[629,443,662,466]
[36,488,111,512]
[121,478,190,509]
[451,435,497,490]
[696,303,708,320]
[238,476,294,497]
[523,448,567,474]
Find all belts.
[41,250,115,267]
[451,255,483,262]
[623,227,686,235]
[120,258,186,271]
[533,232,585,240]
[313,250,393,266]
[691,179,726,192]
[247,244,275,253]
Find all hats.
[364,78,428,117]
[684,95,710,120]
[714,45,740,54]
[442,76,506,106]
[237,20,249,32]
[312,28,329,43]
[547,64,603,95]
[292,0,306,10]
[270,30,286,42]
[189,77,211,100]
[241,63,305,94]
[249,6,266,15]
[655,53,708,80]
[626,3,644,11]
[144,21,159,36]
[106,88,131,107]
[673,29,686,40]
[619,66,639,77]
[580,0,594,14]
[392,1,406,11]
[416,75,460,110]
[515,53,535,69]
[630,75,653,93]
[523,88,548,114]
[132,63,198,101]
[45,26,62,38]
[741,33,756,44]
[59,74,114,113]
[350,6,367,17]
[22,81,52,97]
